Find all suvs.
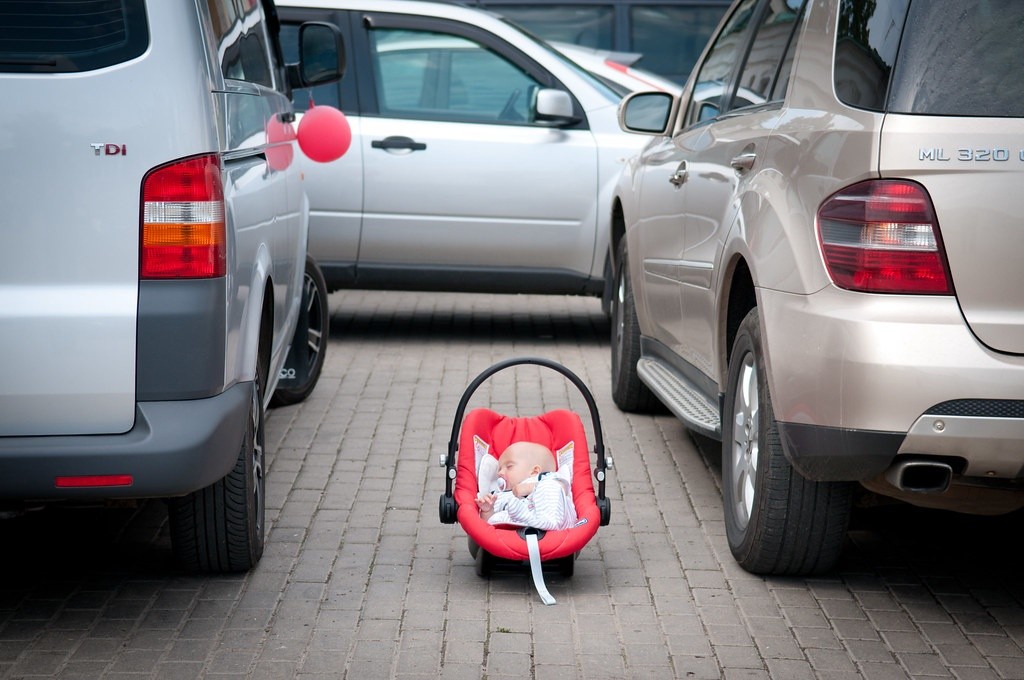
[1,1,345,582]
[603,0,1024,586]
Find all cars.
[372,34,687,129]
[275,2,687,296]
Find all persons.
[474,441,573,531]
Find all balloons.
[264,114,297,173]
[297,99,351,163]
